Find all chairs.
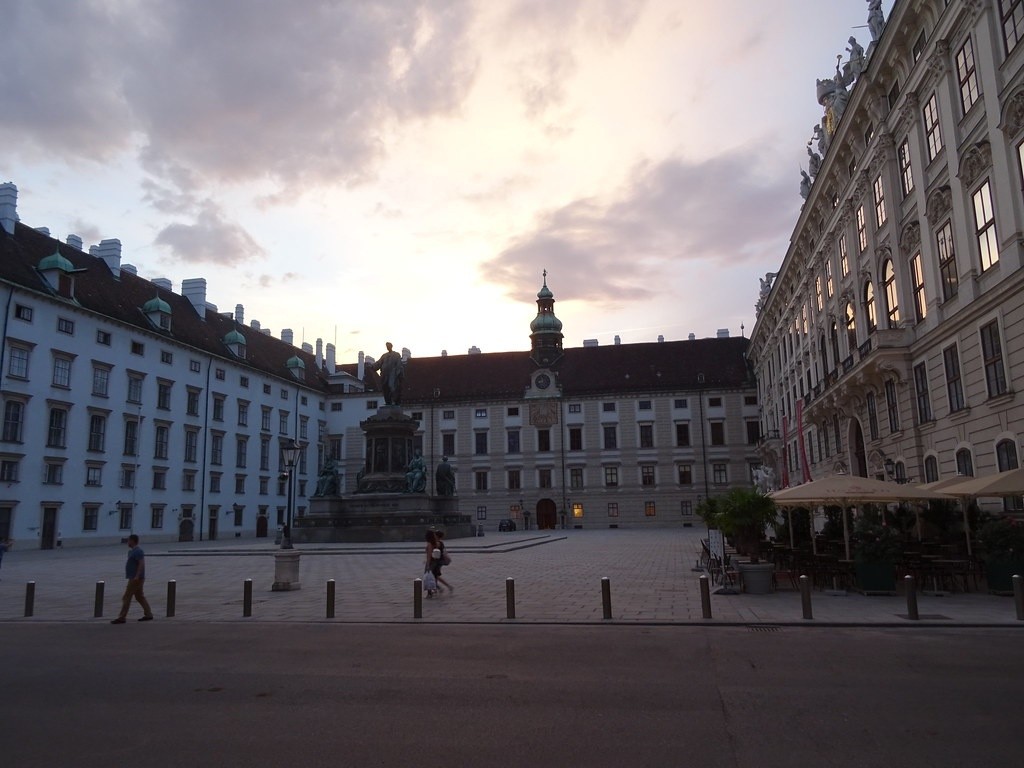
[695,539,743,595]
[773,555,800,592]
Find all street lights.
[270,434,311,591]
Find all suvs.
[498,519,517,532]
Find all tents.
[763,467,1024,591]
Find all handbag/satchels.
[423,570,435,589]
[431,549,440,558]
[442,558,451,566]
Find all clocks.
[535,373,551,391]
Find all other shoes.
[138,616,153,621]
[110,617,127,624]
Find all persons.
[800,170,811,201]
[370,342,405,406]
[423,528,453,600]
[755,278,771,319]
[813,124,828,159]
[110,535,153,624]
[834,65,848,119]
[436,456,456,496]
[281,522,287,538]
[317,448,339,497]
[846,36,865,82]
[406,448,427,494]
[0,535,15,581]
[807,147,819,180]
[866,0,884,41]
